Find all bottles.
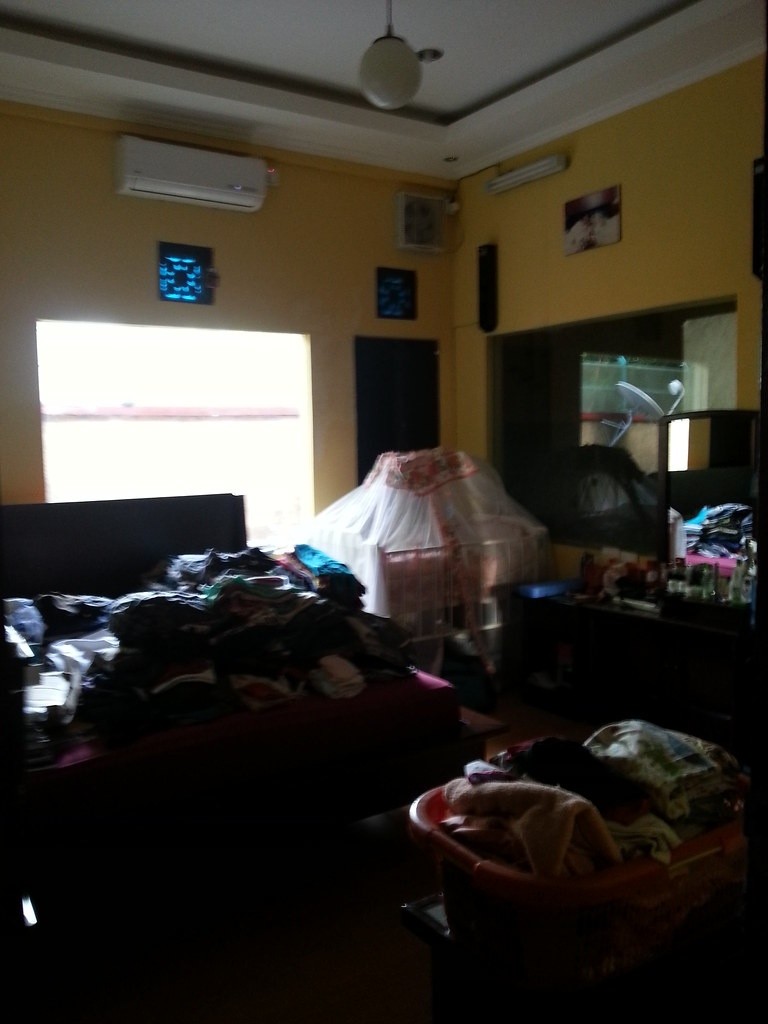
[728,558,743,605]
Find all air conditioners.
[116,134,271,213]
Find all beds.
[302,514,547,637]
[541,502,668,557]
[0,491,511,825]
[667,464,755,580]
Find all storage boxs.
[410,747,753,964]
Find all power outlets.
[445,199,456,216]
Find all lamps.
[360,0,424,111]
[483,153,569,195]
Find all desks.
[488,576,753,717]
[396,867,767,1022]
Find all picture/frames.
[564,184,621,258]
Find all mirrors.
[656,409,759,608]
